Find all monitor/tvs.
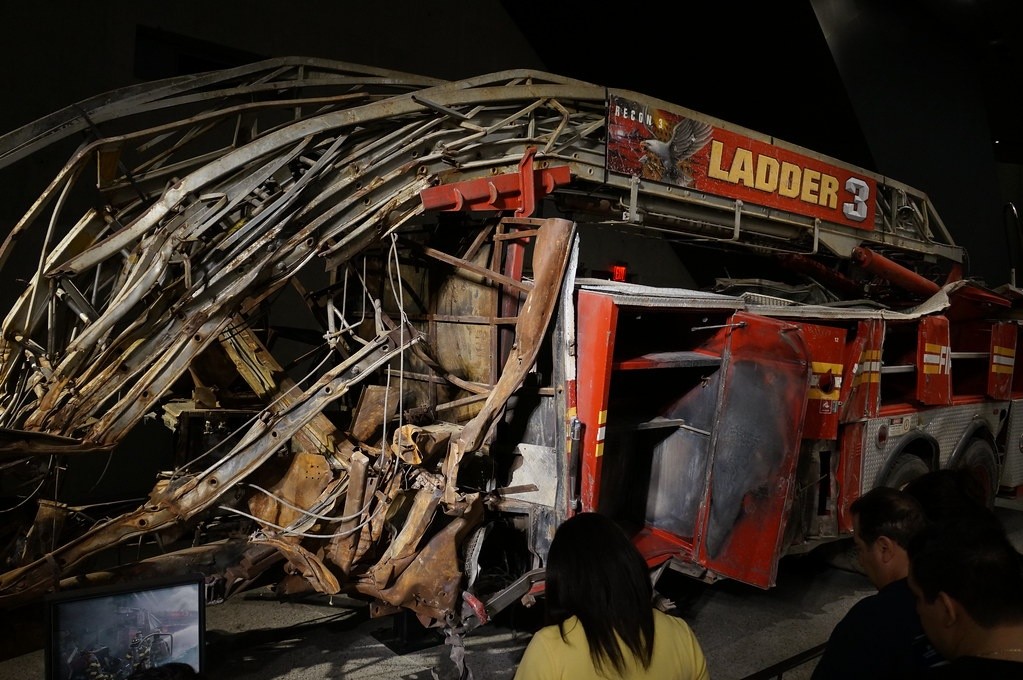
[43,571,207,680]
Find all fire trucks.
[1,59,1023,658]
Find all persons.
[905,504,1023,680]
[512,513,710,680]
[808,486,949,680]
[901,464,983,540]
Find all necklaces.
[975,647,1022,657]
